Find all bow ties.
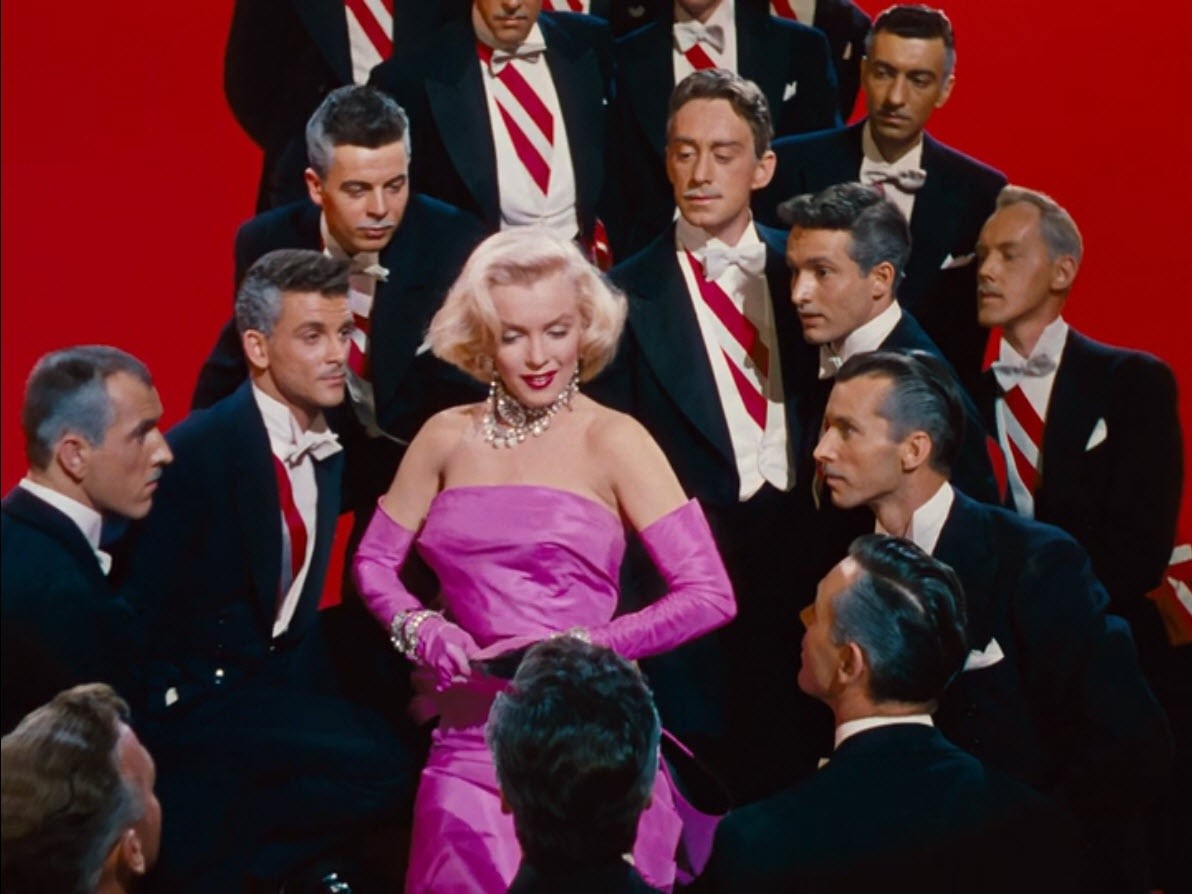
[95,549,112,576]
[490,43,547,75]
[672,21,726,54]
[861,156,926,191]
[991,355,1055,392]
[817,345,846,378]
[325,245,393,284]
[286,434,343,467]
[702,242,766,281]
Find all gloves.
[471,497,736,661]
[353,495,490,693]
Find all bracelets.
[404,608,443,661]
[389,611,412,651]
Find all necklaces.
[480,384,576,449]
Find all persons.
[690,532,1058,893]
[349,224,736,894]
[0,0,1192,894]
[0,682,163,894]
[485,637,666,894]
